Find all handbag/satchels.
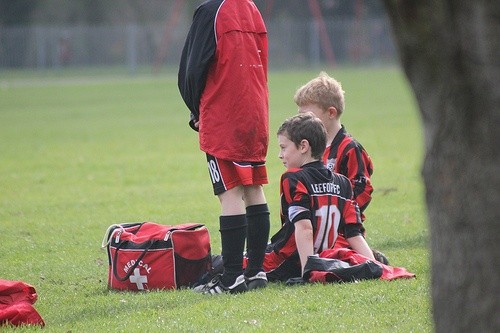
[100,221,211,292]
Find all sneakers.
[185,271,247,296]
[243,269,267,290]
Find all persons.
[295,73,372,238]
[179,1,269,295]
[211,114,377,282]
